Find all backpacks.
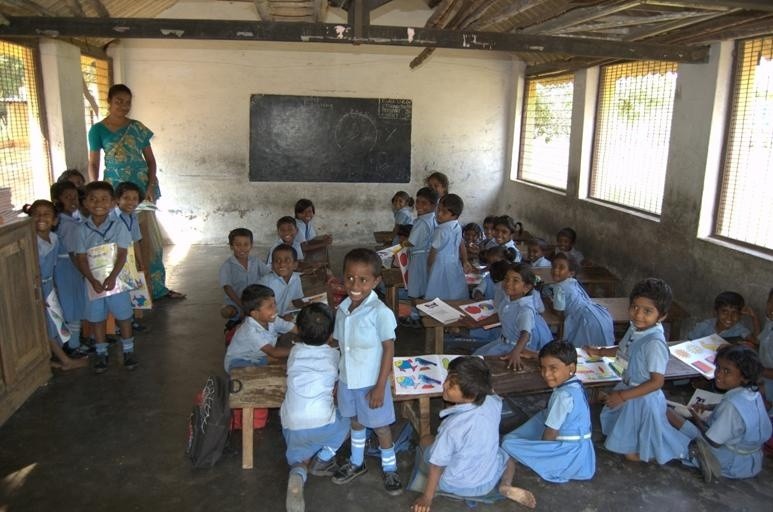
[187,371,241,470]
[366,418,416,456]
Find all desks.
[268,234,341,364]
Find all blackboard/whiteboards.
[249,94,412,183]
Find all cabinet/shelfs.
[0,218,55,428]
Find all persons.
[501,339,597,484]
[88,84,189,302]
[221,199,315,373]
[21,170,146,375]
[279,302,351,512]
[598,277,721,485]
[330,248,404,496]
[461,215,615,373]
[391,171,472,333]
[666,286,773,481]
[408,355,536,512]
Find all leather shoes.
[224,320,240,330]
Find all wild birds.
[418,374,441,385]
[414,357,437,366]
[442,358,450,371]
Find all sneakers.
[52,289,186,373]
[689,437,720,483]
[286,456,403,512]
